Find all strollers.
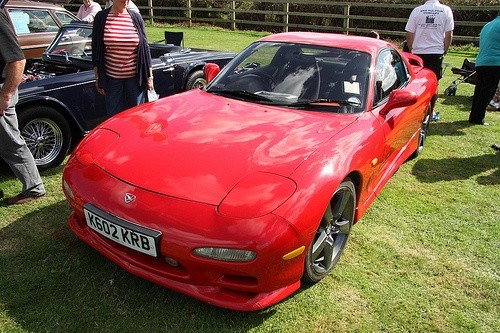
[443,57,476,97]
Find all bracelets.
[95,76,99,80]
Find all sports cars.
[14,22,239,172]
[61,31,439,311]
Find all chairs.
[333,56,373,105]
[276,57,320,101]
[150,46,191,59]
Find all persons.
[469,12,500,125]
[405,0,455,120]
[0,0,46,204]
[76,0,141,24]
[92,0,155,118]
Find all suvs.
[3,2,92,76]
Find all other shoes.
[432,113,439,120]
[475,120,489,125]
[9,194,42,204]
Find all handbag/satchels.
[137,88,159,106]
